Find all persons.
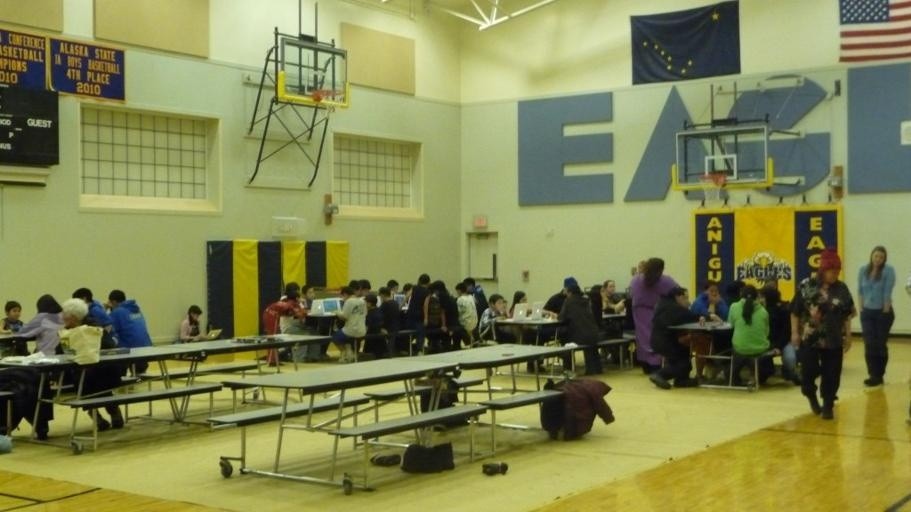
[264,256,801,390]
[55,299,124,429]
[1,301,23,332]
[180,305,201,340]
[107,289,152,373]
[788,250,855,419]
[73,287,112,334]
[857,245,893,385]
[19,294,62,356]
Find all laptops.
[393,295,408,310]
[321,298,341,316]
[307,298,323,316]
[200,328,223,341]
[527,303,543,321]
[505,303,528,322]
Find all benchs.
[207,393,373,478]
[58,383,223,454]
[0,377,139,434]
[480,389,563,454]
[733,348,780,391]
[139,360,267,417]
[550,336,631,380]
[353,329,417,363]
[327,404,489,496]
[363,376,488,449]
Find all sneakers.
[674,378,697,387]
[112,409,123,427]
[823,404,833,418]
[812,401,821,413]
[650,375,671,389]
[97,417,109,430]
[864,377,883,386]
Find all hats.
[364,295,376,303]
[820,250,841,271]
[565,278,576,288]
[188,306,201,314]
[37,295,62,313]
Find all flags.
[838,0,910,70]
[630,1,741,85]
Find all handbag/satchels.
[57,325,102,364]
[782,339,804,385]
